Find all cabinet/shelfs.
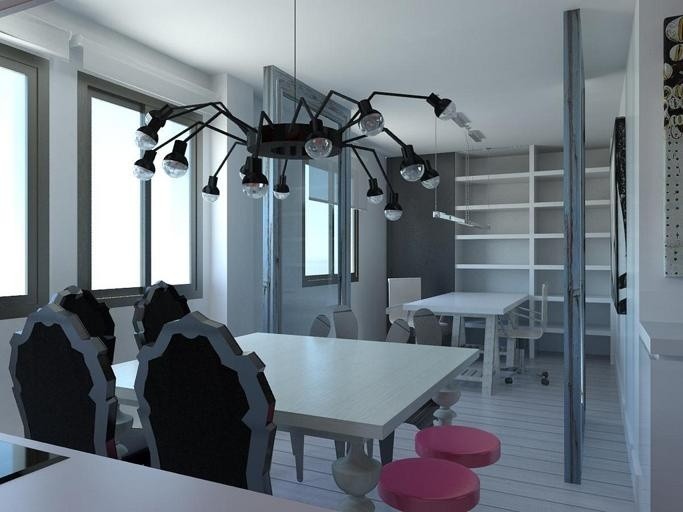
[454,145,615,362]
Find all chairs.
[9,302,119,459]
[55,284,116,367]
[135,278,189,349]
[387,276,451,330]
[134,312,275,498]
[309,305,444,346]
[501,282,552,387]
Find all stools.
[416,424,501,468]
[377,456,481,512]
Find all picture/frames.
[609,116,627,315]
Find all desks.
[113,331,481,512]
[1,431,325,510]
[402,292,531,399]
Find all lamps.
[434,101,491,230]
[134,0,457,221]
